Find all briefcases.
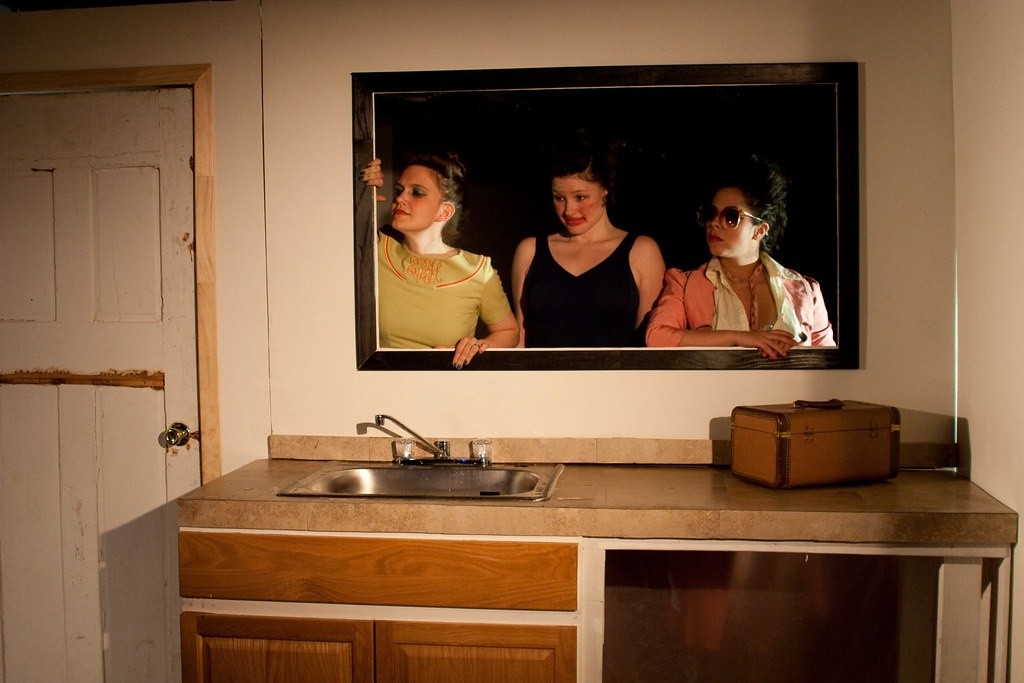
[731,399,900,490]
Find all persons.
[646,162,837,360]
[513,154,666,349]
[359,150,521,369]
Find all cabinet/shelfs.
[174,530,581,683]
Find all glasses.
[695,203,765,231]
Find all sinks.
[274,459,565,502]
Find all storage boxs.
[728,395,901,489]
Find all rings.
[474,343,481,348]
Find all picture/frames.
[352,62,861,370]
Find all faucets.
[374,414,492,470]
